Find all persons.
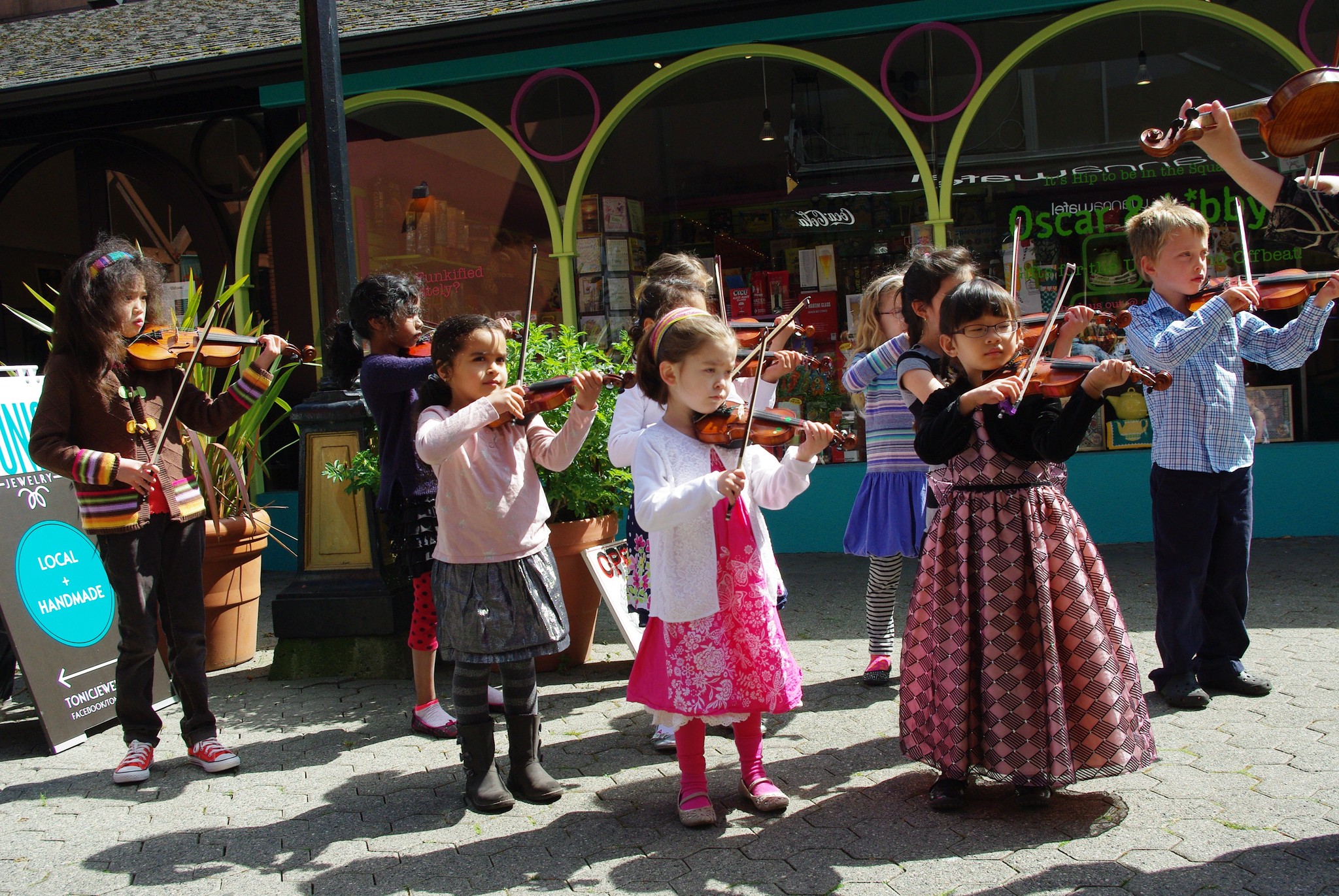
[346,271,512,739]
[1179,98,1339,274]
[595,252,1092,831]
[26,235,282,782]
[895,274,1159,812]
[1115,193,1339,710]
[413,314,602,813]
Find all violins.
[1138,66,1339,161]
[128,322,317,373]
[726,318,815,346]
[487,371,640,430]
[1182,270,1339,317]
[405,328,531,358]
[731,351,834,381]
[981,352,1170,399]
[691,399,858,453]
[1017,306,1132,349]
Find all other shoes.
[1150,666,1210,706]
[1015,780,1053,807]
[675,788,716,825]
[862,661,890,685]
[1195,666,1272,696]
[650,725,677,751]
[739,777,790,812]
[929,773,969,811]
[720,719,768,737]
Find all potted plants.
[318,320,639,674]
[89,272,275,677]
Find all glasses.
[877,309,903,319]
[953,320,1021,338]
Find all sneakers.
[113,739,155,782]
[187,737,240,772]
[486,698,505,713]
[411,709,459,741]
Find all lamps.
[1132,49,1154,89]
[760,107,777,141]
[410,180,432,199]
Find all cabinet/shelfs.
[357,252,481,326]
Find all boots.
[455,713,516,810]
[505,712,564,801]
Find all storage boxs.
[1059,391,1158,452]
[698,268,878,466]
[995,230,1073,323]
[1238,384,1295,444]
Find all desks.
[757,463,868,554]
[1064,440,1339,547]
